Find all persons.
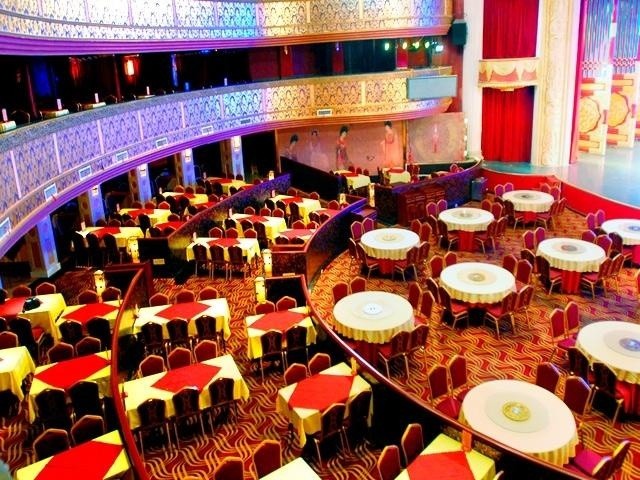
[283,134,301,162]
[380,121,399,167]
[335,125,352,171]
[307,128,330,173]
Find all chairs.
[493,181,526,234]
[137,376,239,460]
[560,439,633,479]
[140,338,220,378]
[580,252,626,301]
[473,199,510,254]
[75,286,122,304]
[394,216,433,283]
[376,321,430,381]
[425,275,471,332]
[148,286,221,308]
[31,414,104,462]
[482,284,536,338]
[522,227,548,252]
[10,80,246,134]
[567,345,629,430]
[59,316,112,346]
[405,281,435,342]
[426,354,472,421]
[375,423,424,480]
[34,379,106,427]
[347,217,382,280]
[533,181,566,230]
[191,243,248,283]
[426,198,462,252]
[258,324,309,383]
[312,389,372,472]
[534,361,591,450]
[327,164,468,194]
[0,282,58,300]
[520,247,562,297]
[68,172,353,267]
[502,253,534,292]
[0,316,48,361]
[44,337,104,365]
[430,250,458,279]
[253,294,299,316]
[586,209,634,268]
[140,315,218,350]
[580,230,612,257]
[211,438,284,480]
[331,275,367,305]
[546,301,581,363]
[284,352,333,387]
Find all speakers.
[453,19,467,45]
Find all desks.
[394,432,495,480]
[258,455,322,480]
[275,359,375,448]
[439,262,517,304]
[501,189,555,212]
[333,290,414,344]
[27,349,115,425]
[600,218,640,246]
[243,305,318,361]
[0,344,36,404]
[14,429,131,480]
[132,298,233,353]
[118,354,249,429]
[52,297,125,342]
[439,205,493,232]
[458,378,580,467]
[574,320,639,385]
[360,227,421,261]
[0,120,17,133]
[0,291,68,343]
